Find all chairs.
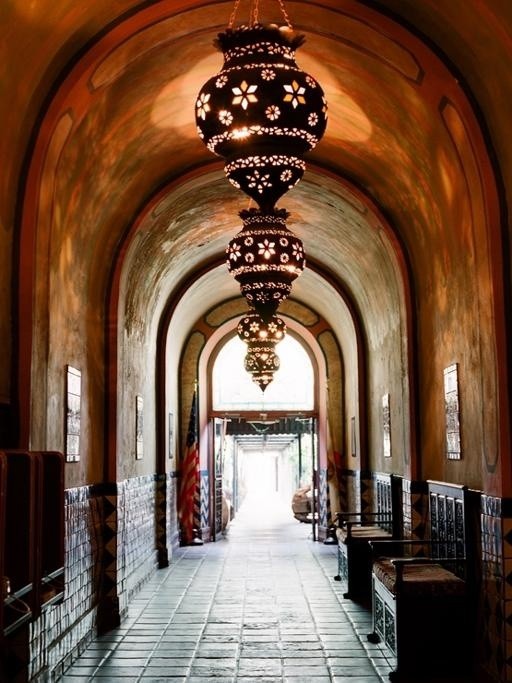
[335,470,475,682]
